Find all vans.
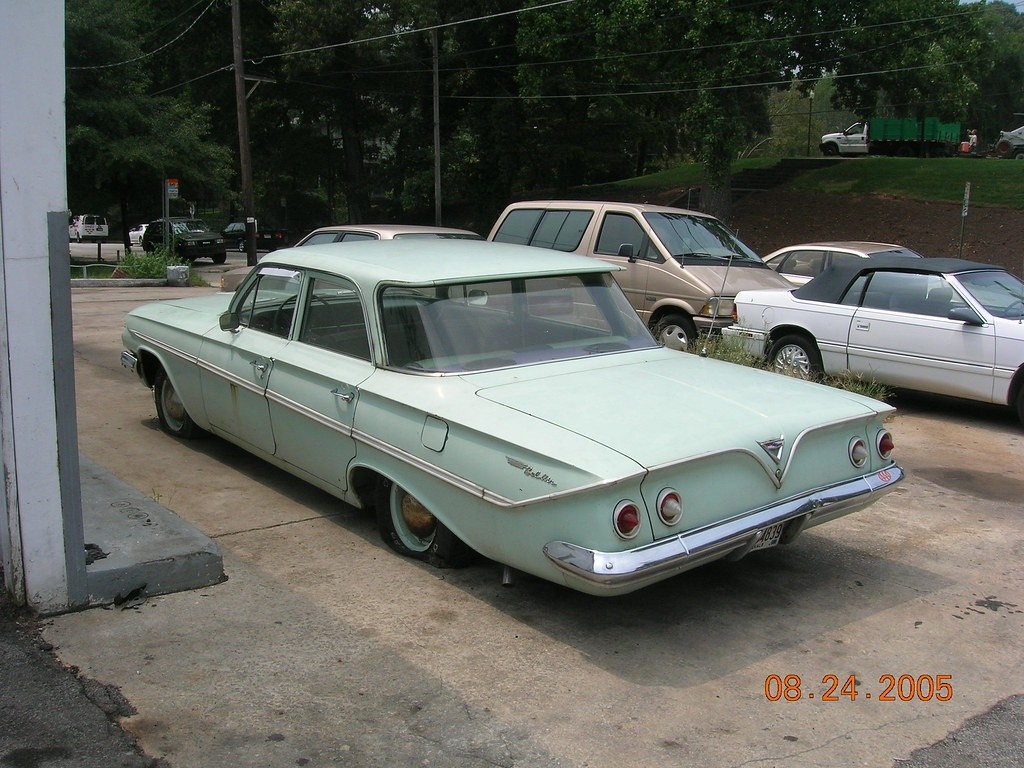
[484,200,798,357]
[68,214,108,243]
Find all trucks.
[819,116,961,157]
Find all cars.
[219,222,284,253]
[120,239,904,598]
[128,224,150,247]
[759,240,1023,316]
[999,126,1024,160]
[720,258,1024,431]
[219,224,486,292]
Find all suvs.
[142,216,227,266]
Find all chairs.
[923,287,956,317]
[809,258,822,276]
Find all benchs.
[403,334,628,370]
[308,316,474,355]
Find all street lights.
[807,90,814,158]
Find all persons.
[966,129,978,152]
